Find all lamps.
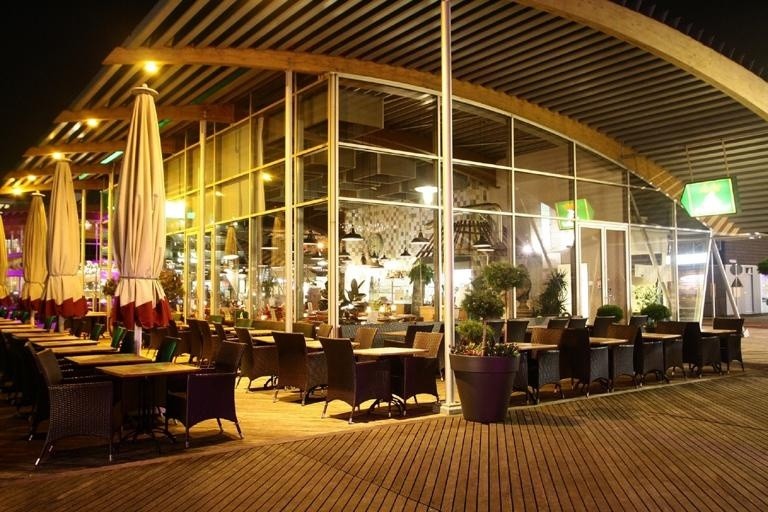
[414,161,437,204]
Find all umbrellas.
[0,211,11,318]
[20,190,47,324]
[270,206,284,269]
[43,158,88,331]
[222,224,241,288]
[109,84,167,357]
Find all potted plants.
[640,302,672,333]
[450,261,530,423]
